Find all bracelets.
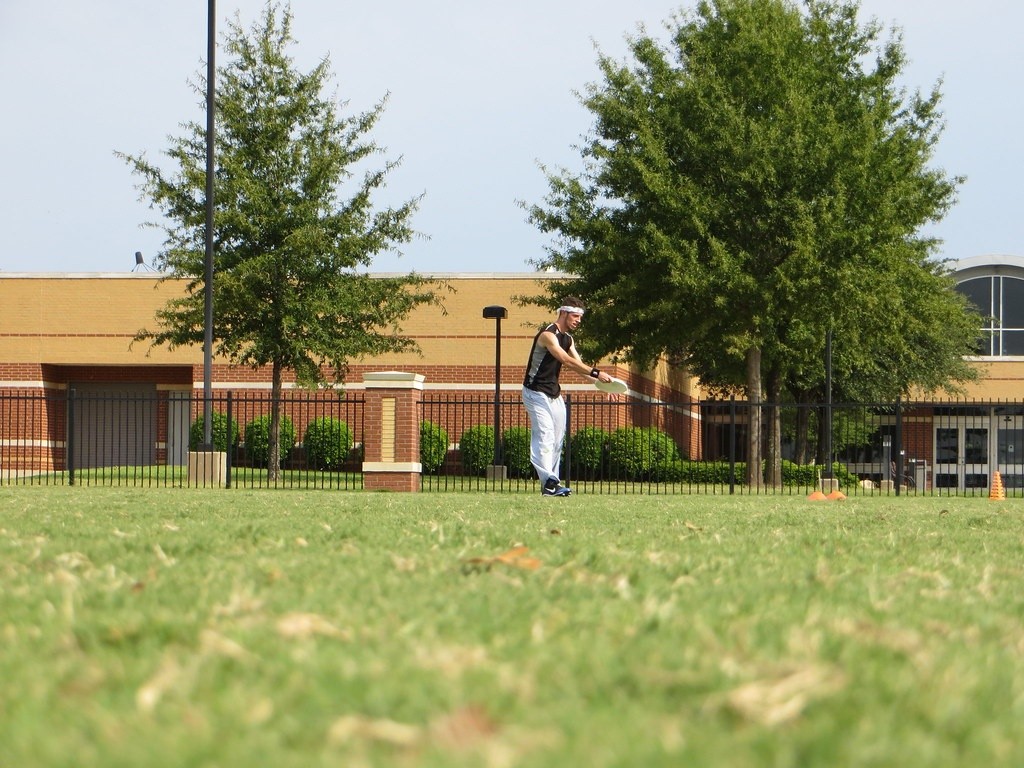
[590,367,600,378]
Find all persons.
[522,296,612,497]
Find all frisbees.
[596,378,626,392]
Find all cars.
[865,462,916,488]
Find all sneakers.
[542,476,571,497]
